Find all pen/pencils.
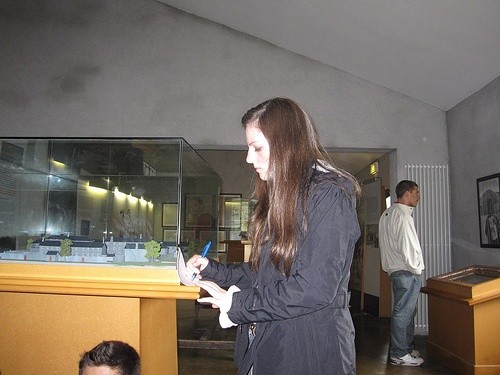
[190,241,212,283]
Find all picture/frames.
[476,172,500,249]
[161,202,178,226]
[163,228,177,243]
[183,192,216,228]
[180,229,197,243]
[219,193,241,231]
[197,228,229,253]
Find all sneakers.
[388,350,423,366]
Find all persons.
[184,96,363,375]
[78,340,142,375]
[188,195,215,324]
[377,180,425,367]
